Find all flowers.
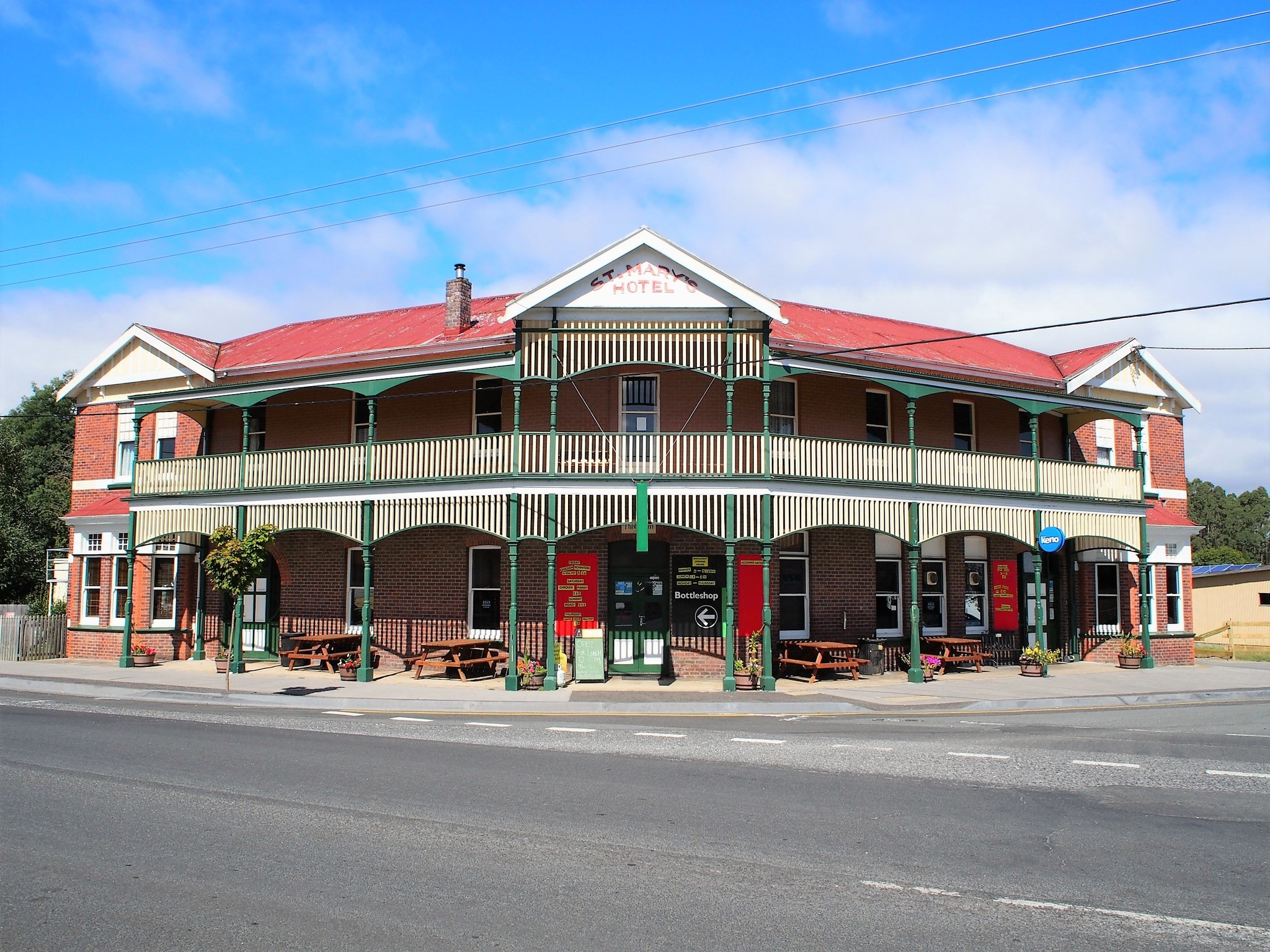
[1104,630,1149,656]
[216,648,236,659]
[1019,640,1061,666]
[131,646,156,654]
[336,654,362,668]
[919,654,941,669]
[515,633,546,685]
[901,651,911,668]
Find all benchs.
[278,648,377,675]
[778,656,870,683]
[403,654,509,683]
[911,648,993,677]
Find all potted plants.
[734,630,762,690]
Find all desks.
[415,639,494,682]
[923,638,982,675]
[777,640,858,684]
[288,634,363,673]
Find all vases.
[215,658,232,673]
[1021,661,1044,677]
[131,653,155,667]
[521,673,546,690]
[1117,655,1141,668]
[338,665,357,681]
[922,669,934,681]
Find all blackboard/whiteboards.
[575,638,605,680]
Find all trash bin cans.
[279,631,307,667]
[857,637,887,675]
